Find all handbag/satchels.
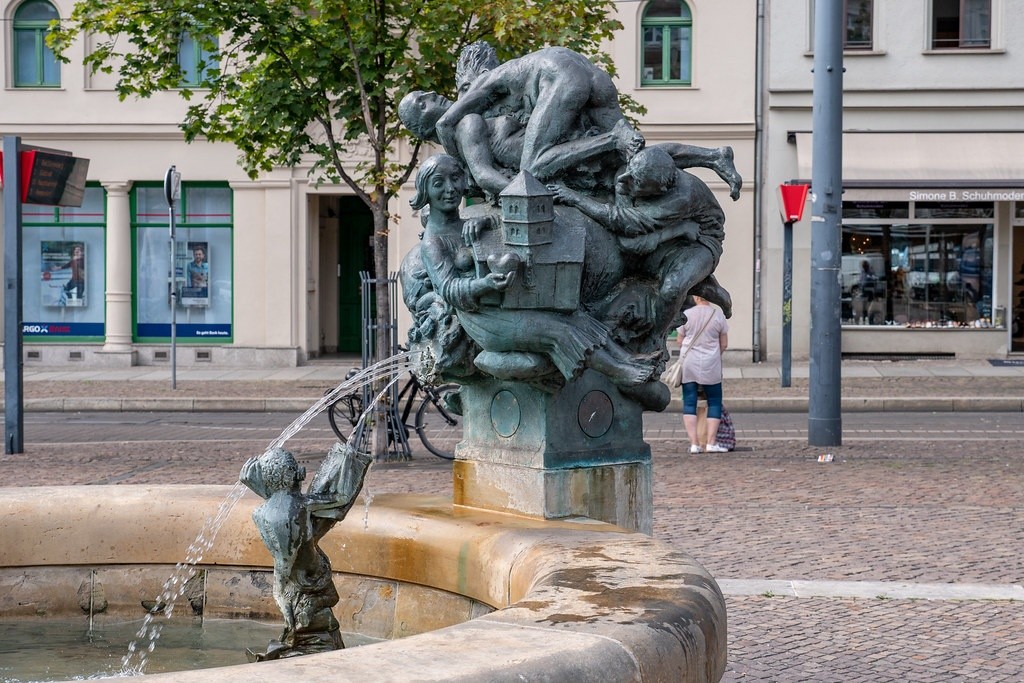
[660,359,682,388]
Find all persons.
[399,39,744,388]
[661,282,730,453]
[50,245,85,300]
[242,449,358,633]
[186,246,208,289]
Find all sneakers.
[705,443,728,452]
[691,445,704,453]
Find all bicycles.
[324,344,463,459]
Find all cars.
[840,236,993,305]
